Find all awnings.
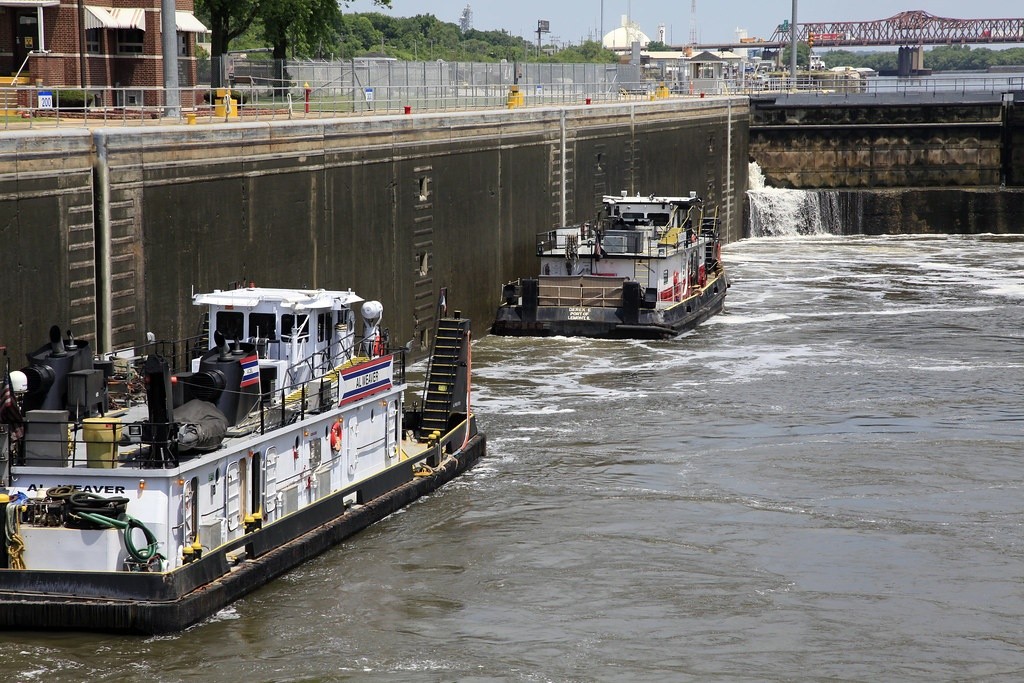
[83,4,207,34]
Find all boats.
[0,283,488,634]
[490,191,733,340]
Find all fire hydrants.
[303,82,312,113]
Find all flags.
[0,361,24,441]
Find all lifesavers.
[374,335,384,357]
[674,271,679,285]
[331,424,343,451]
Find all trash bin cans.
[509,86,523,106]
[214,90,237,116]
[656,82,669,97]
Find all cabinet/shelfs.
[106,355,144,405]
